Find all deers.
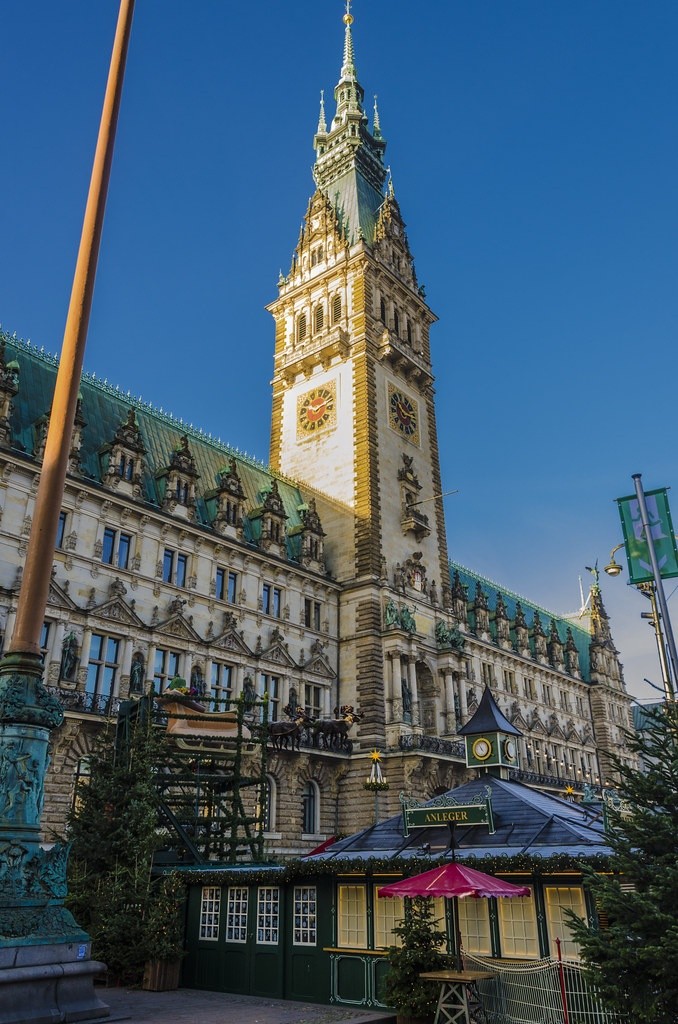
[267,705,365,752]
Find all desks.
[420,969,497,1024]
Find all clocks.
[501,736,517,764]
[470,735,495,763]
[383,375,424,451]
[292,373,342,444]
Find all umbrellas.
[377,863,532,971]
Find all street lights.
[603,542,674,716]
